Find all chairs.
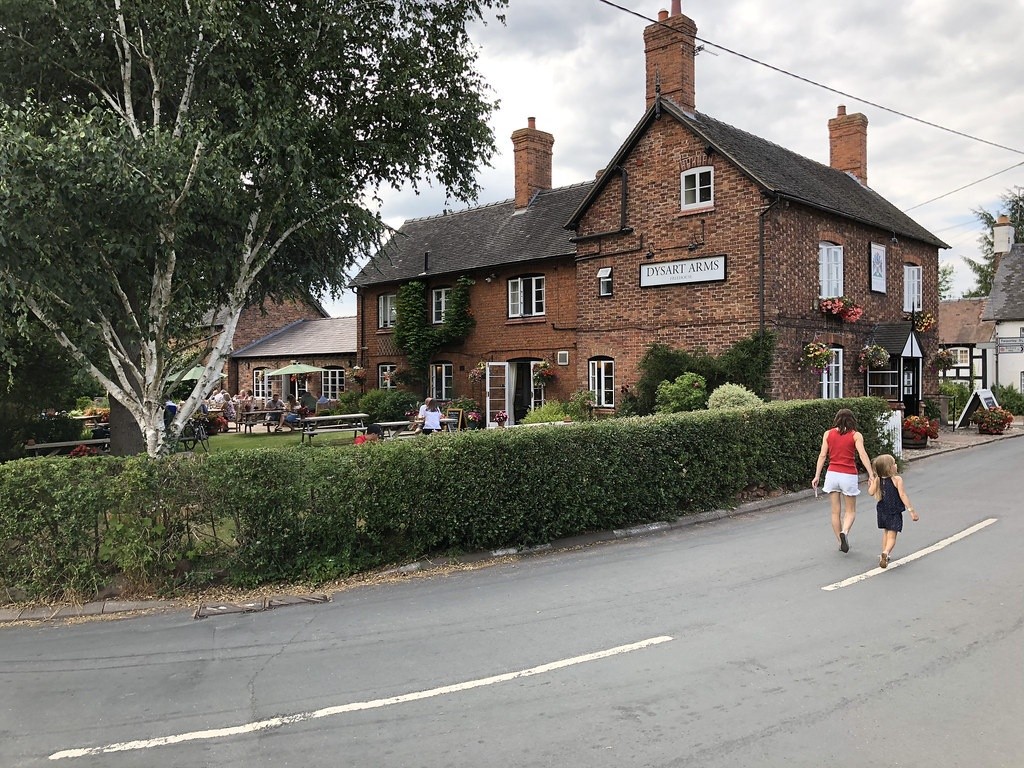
[311,401,338,417]
[227,407,239,432]
[204,399,224,409]
[255,398,263,411]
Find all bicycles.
[183,412,211,453]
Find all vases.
[209,428,218,435]
[498,421,505,427]
[409,414,416,421]
[468,420,478,428]
[977,423,995,435]
[481,374,486,379]
[902,430,928,449]
[301,415,306,420]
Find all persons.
[354,424,383,445]
[422,399,441,434]
[209,390,227,403]
[221,393,235,432]
[177,396,209,427]
[868,455,919,568]
[299,391,327,415]
[235,390,255,421]
[284,397,301,431]
[266,394,286,429]
[415,398,446,434]
[812,409,874,553]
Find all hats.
[368,424,384,436]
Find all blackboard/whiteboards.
[955,389,1008,430]
[446,409,468,432]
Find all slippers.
[276,428,283,431]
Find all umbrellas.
[264,362,329,400]
[163,363,228,381]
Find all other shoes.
[879,552,890,567]
[839,531,849,553]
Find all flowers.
[493,410,508,423]
[858,344,891,374]
[968,406,1014,434]
[205,412,228,430]
[904,312,936,333]
[796,342,839,375]
[344,366,367,385]
[441,394,482,422]
[902,415,940,442]
[297,406,310,415]
[468,361,487,383]
[405,401,425,416]
[290,373,314,383]
[532,353,560,386]
[255,368,274,381]
[928,350,959,374]
[383,368,418,385]
[819,297,864,324]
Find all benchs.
[239,421,280,424]
[85,422,110,426]
[293,423,361,430]
[303,427,368,434]
[384,430,418,438]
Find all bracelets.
[908,507,913,511]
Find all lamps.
[646,242,663,259]
[688,231,705,251]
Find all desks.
[25,438,111,458]
[374,419,458,438]
[208,409,224,432]
[239,410,291,434]
[72,415,101,428]
[295,413,369,447]
